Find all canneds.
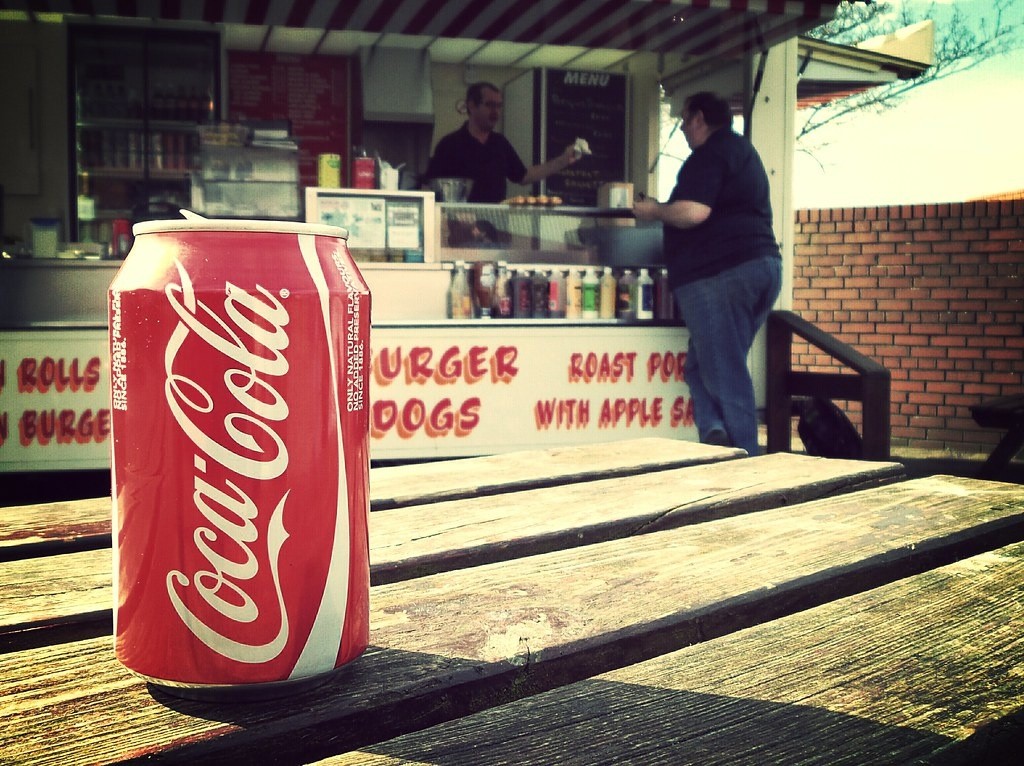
[352,157,376,189]
[104,208,370,698]
[101,128,197,173]
[317,153,340,188]
[79,222,112,243]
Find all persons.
[633,91,783,456]
[418,81,582,249]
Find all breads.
[499,195,563,206]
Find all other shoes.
[706,430,729,446]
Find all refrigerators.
[62,13,230,247]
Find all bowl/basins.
[428,179,473,202]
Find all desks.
[0,436,1024,766]
[968,393,1024,484]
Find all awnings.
[0,0,842,86]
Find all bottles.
[450,259,675,321]
[82,85,214,120]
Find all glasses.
[478,100,503,108]
